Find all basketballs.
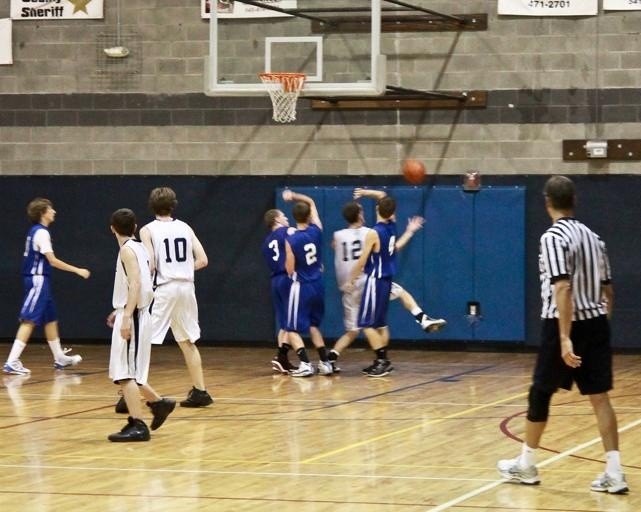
[403,158,427,186]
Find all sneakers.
[417,314,447,334]
[271,353,292,373]
[179,385,214,407]
[589,471,628,493]
[3,360,32,373]
[272,357,298,372]
[364,360,395,378]
[110,415,151,442]
[315,360,334,375]
[362,362,376,374]
[146,399,177,431]
[53,348,83,370]
[329,356,341,373]
[495,457,539,484]
[289,361,315,376]
[115,396,129,413]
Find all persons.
[2,200,91,375]
[282,185,332,379]
[263,210,323,374]
[496,176,629,493]
[115,186,213,412]
[326,203,446,370]
[349,187,398,377]
[109,208,175,443]
